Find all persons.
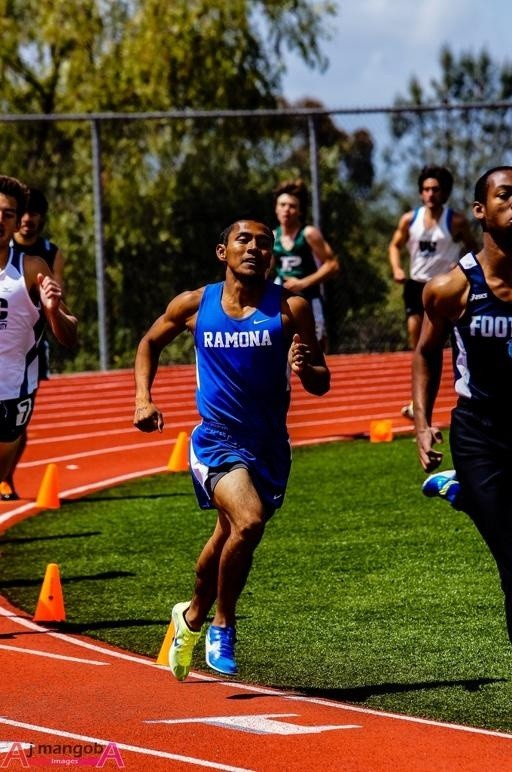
[0,175,78,456]
[265,178,341,342]
[0,188,64,501]
[412,165,512,642]
[389,167,481,443]
[133,217,331,682]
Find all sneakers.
[205,624,238,676]
[169,601,205,681]
[421,469,459,503]
